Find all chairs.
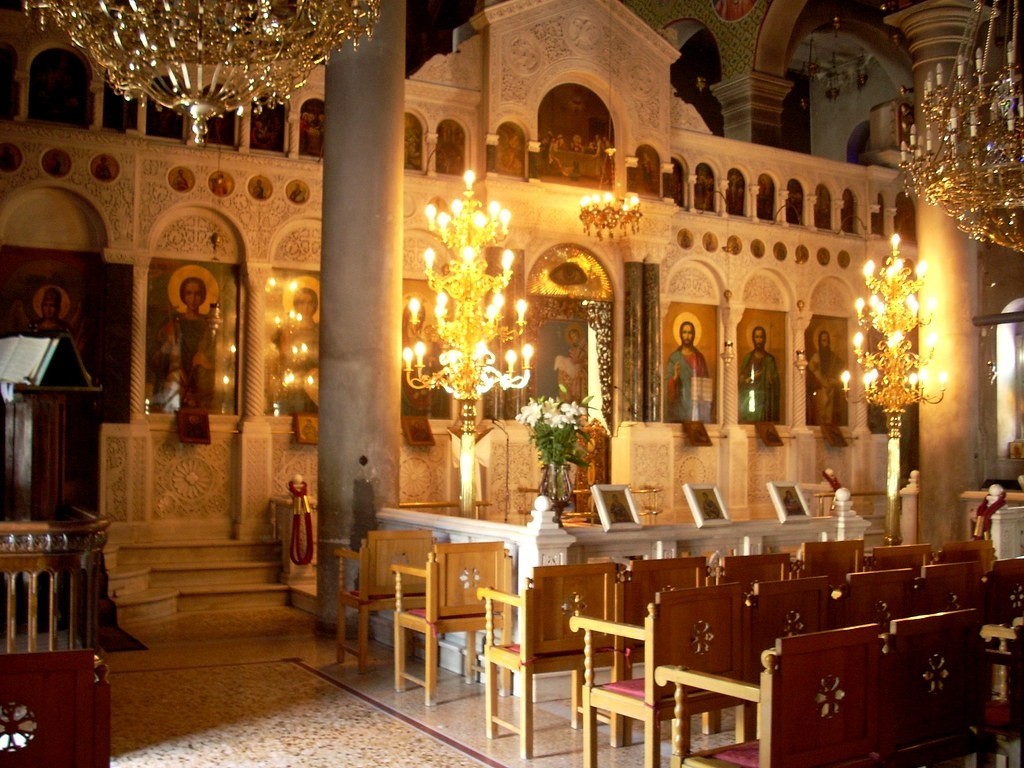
[332,530,440,675]
[476,539,1023,768]
[387,539,514,706]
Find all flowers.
[514,384,598,468]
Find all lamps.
[840,231,952,544]
[695,76,705,92]
[401,166,536,519]
[898,1,1024,257]
[799,16,868,110]
[577,147,642,243]
[19,0,381,150]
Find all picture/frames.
[821,423,848,449]
[402,414,436,448]
[177,408,211,445]
[590,483,643,532]
[681,483,732,529]
[766,480,813,524]
[683,420,712,449]
[754,421,783,447]
[292,412,319,446]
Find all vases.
[537,462,574,528]
